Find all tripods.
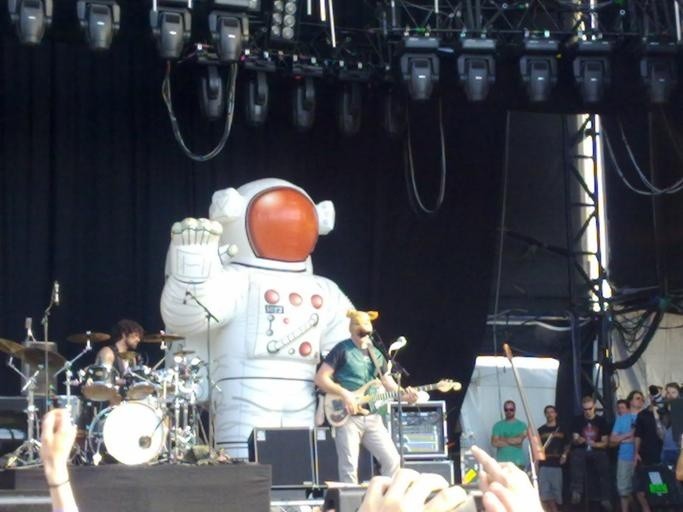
[4,320,233,469]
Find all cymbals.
[172,350,194,356]
[1,339,29,358]
[66,333,110,342]
[143,334,184,343]
[22,349,66,369]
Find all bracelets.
[47,479,70,488]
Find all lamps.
[146,0,196,64]
[202,6,252,67]
[396,34,445,102]
[566,36,625,109]
[257,2,306,54]
[452,34,501,107]
[514,34,564,108]
[3,1,56,52]
[72,0,125,55]
[192,70,403,134]
[634,36,682,112]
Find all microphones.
[358,329,371,337]
[54,282,60,307]
[183,289,188,304]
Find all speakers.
[312,427,374,490]
[246,426,316,490]
[322,487,368,512]
[401,460,455,493]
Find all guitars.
[324,377,462,425]
[502,343,546,462]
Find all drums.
[77,364,119,403]
[151,369,180,395]
[123,367,155,400]
[88,401,165,465]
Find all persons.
[40,408,80,512]
[315,310,418,484]
[357,444,545,512]
[490,401,527,471]
[94,320,144,399]
[537,382,683,512]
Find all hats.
[346,309,378,332]
